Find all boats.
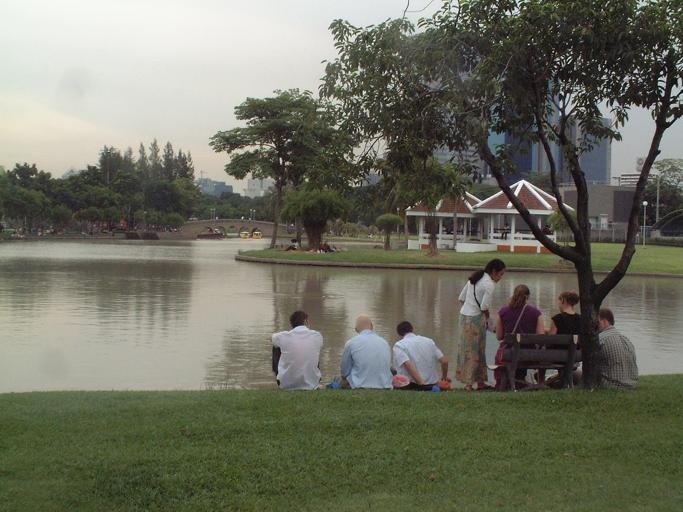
[239,232,250,239]
[251,232,262,239]
[198,232,224,239]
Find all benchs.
[485,332,583,388]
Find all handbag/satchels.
[486,317,496,333]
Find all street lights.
[252,208,256,221]
[641,201,649,247]
[249,208,252,220]
[396,206,401,238]
[210,208,212,219]
[212,208,215,219]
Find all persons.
[391,319,450,391]
[329,313,395,390]
[542,224,550,235]
[570,308,640,390]
[532,289,582,384]
[283,238,349,254]
[500,222,511,240]
[453,258,508,391]
[494,284,545,390]
[269,309,324,390]
